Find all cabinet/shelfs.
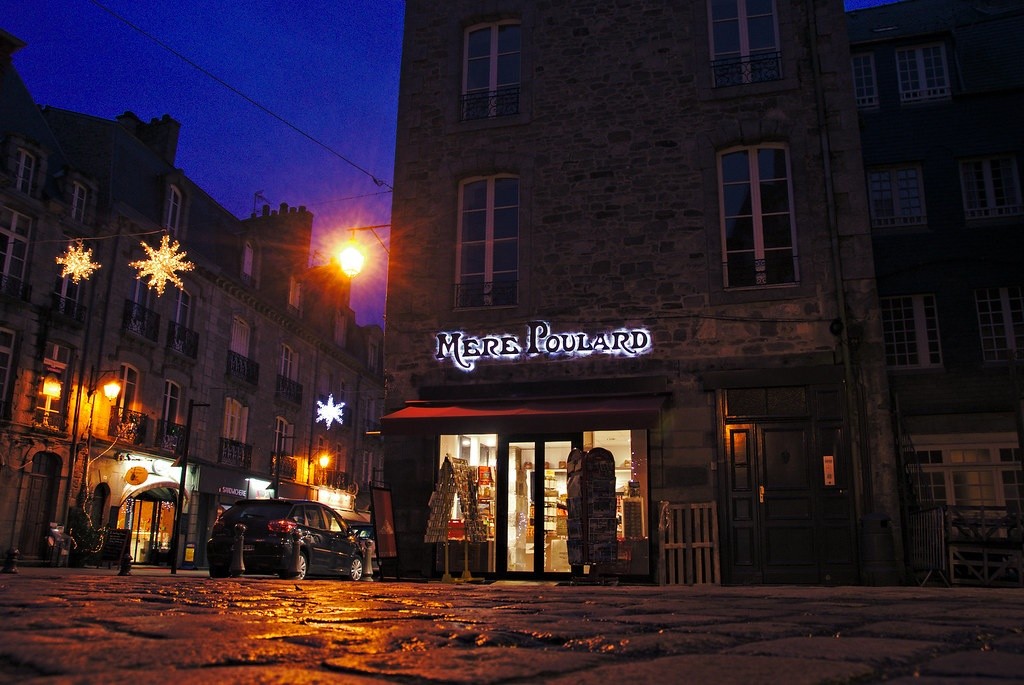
[529,448,647,586]
[424,455,496,583]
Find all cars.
[206,499,364,581]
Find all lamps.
[87,364,123,402]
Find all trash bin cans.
[859,512,899,586]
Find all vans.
[346,521,382,578]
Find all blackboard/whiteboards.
[99,528,130,563]
